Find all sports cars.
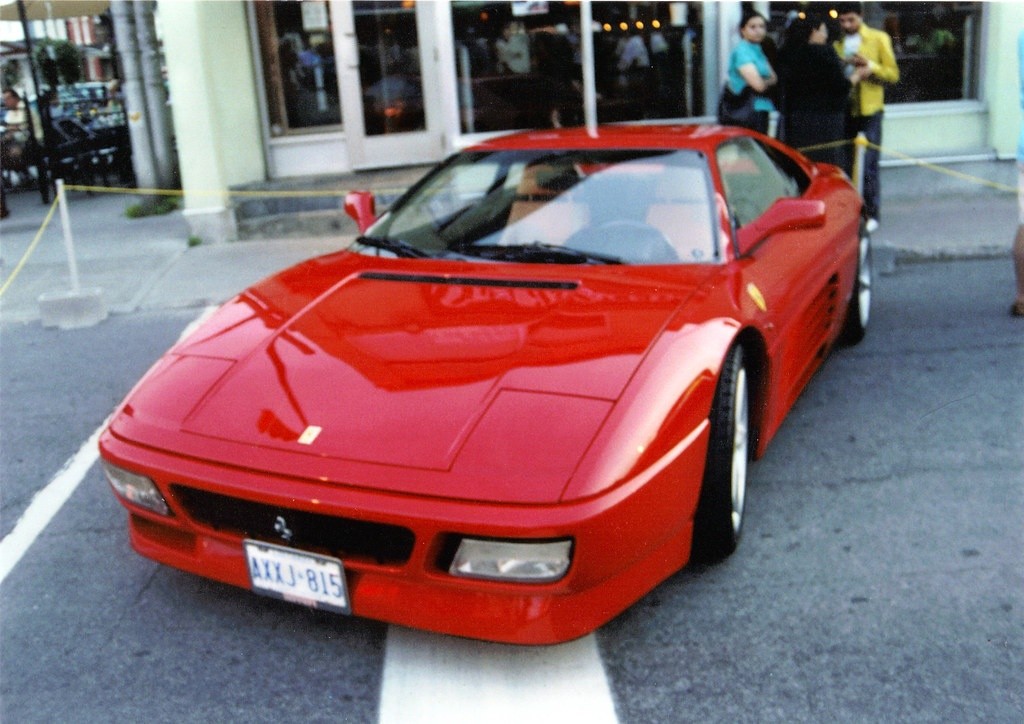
[99,122,871,645]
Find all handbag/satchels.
[718,84,769,133]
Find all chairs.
[499,163,591,252]
[645,167,714,262]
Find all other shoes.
[866,218,878,232]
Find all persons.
[830,2,901,233]
[1007,30,1024,319]
[722,12,778,138]
[778,10,873,180]
[277,20,676,128]
[2,89,44,187]
